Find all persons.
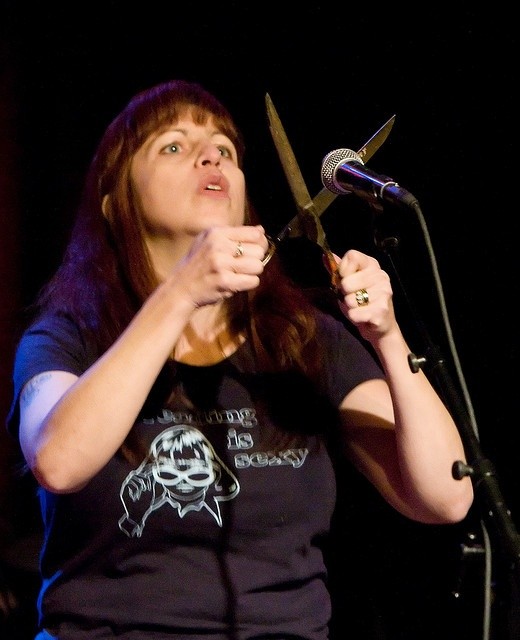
[0,80,475,640]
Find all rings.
[235,240,245,257]
[354,288,369,306]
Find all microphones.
[321,149,418,208]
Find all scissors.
[263,91,395,301]
